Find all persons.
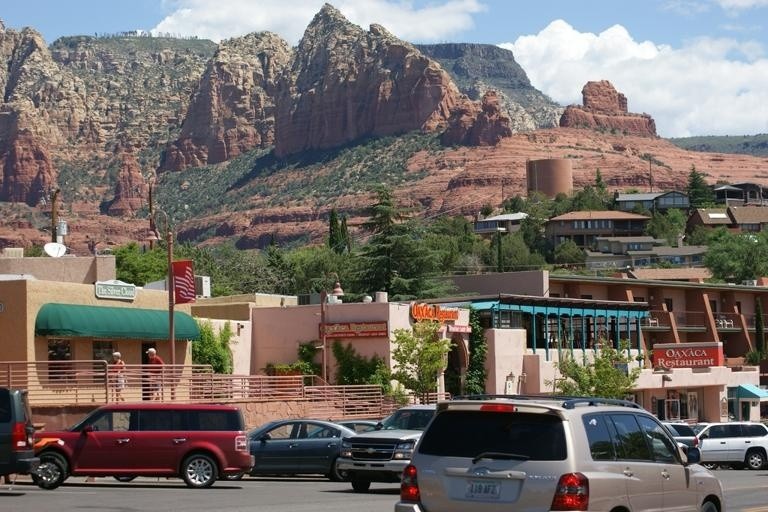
[146,348,165,400]
[111,351,127,402]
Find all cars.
[219,420,380,482]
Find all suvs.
[28,403,254,489]
[337,405,436,492]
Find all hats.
[145,347,156,354]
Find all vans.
[0,385,39,476]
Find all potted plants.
[274,360,311,396]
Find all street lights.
[146,210,176,402]
[52,190,68,243]
[321,272,344,402]
